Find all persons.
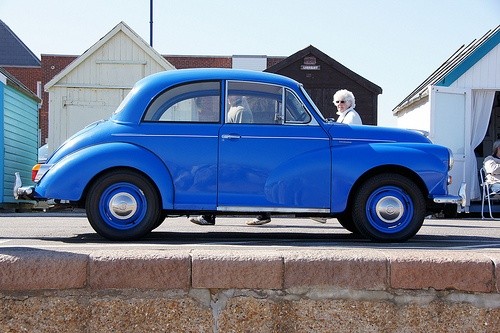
[310,89,362,224]
[188,95,272,226]
[482,140,500,193]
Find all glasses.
[335,100,347,103]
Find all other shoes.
[309,217,326,223]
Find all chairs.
[480,168,500,221]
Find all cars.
[31,68,463,243]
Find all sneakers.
[190,215,212,224]
[246,217,271,224]
